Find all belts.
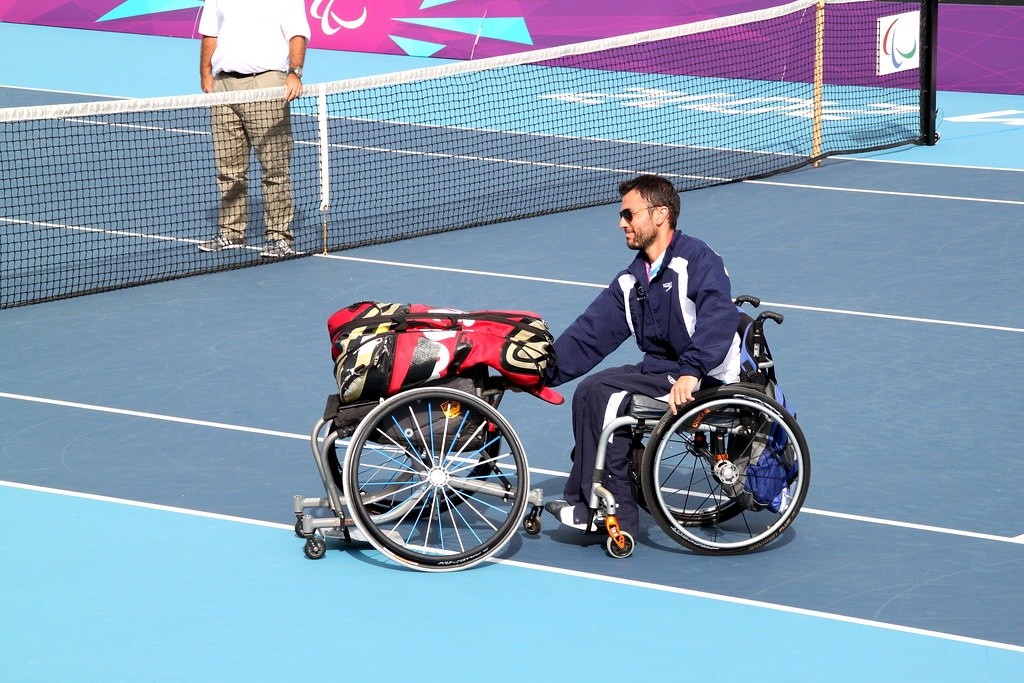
[220,70,271,79]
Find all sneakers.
[198,235,245,252]
[260,239,291,257]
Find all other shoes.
[545,499,571,514]
[554,504,605,531]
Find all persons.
[486,174,742,540]
[197,0,311,258]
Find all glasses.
[619,205,657,222]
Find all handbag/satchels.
[327,301,565,405]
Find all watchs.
[288,66,303,79]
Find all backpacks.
[736,305,801,515]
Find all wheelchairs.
[293,364,545,573]
[571,295,811,560]
